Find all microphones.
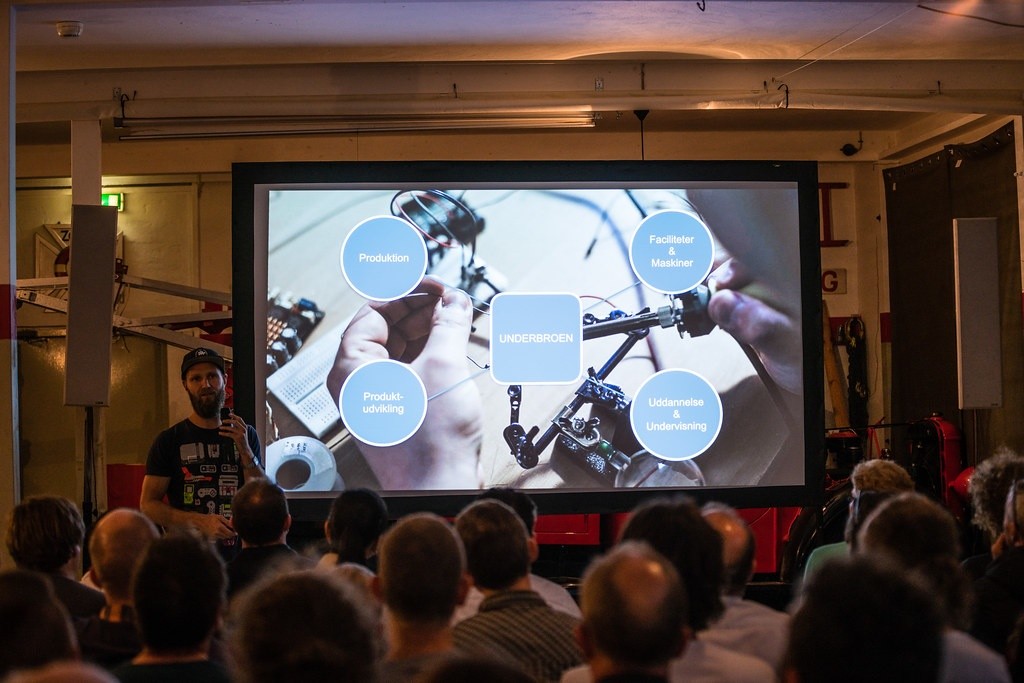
[220,407,235,464]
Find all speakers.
[63,204,118,406]
[953,217,1002,410]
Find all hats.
[181,347,224,380]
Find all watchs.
[248,456,259,468]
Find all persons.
[140,347,267,548]
[0,452,1024,683]
[326,249,802,490]
